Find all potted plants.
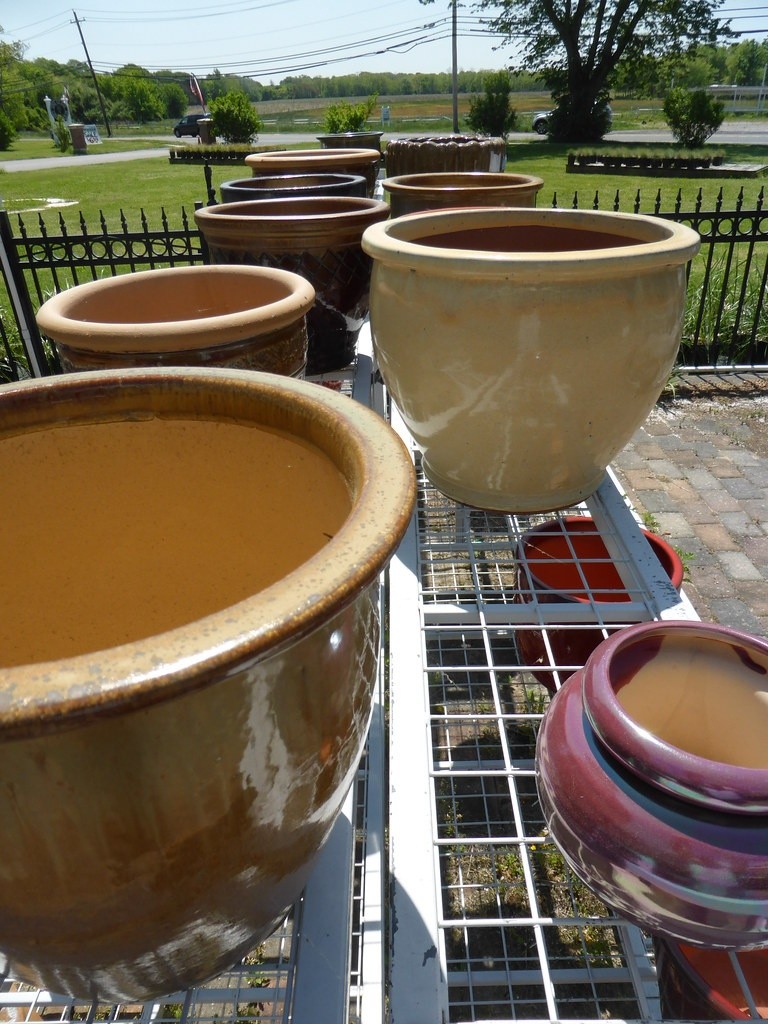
[170,147,175,158]
[566,148,577,165]
[712,150,721,166]
[720,150,725,165]
[176,143,286,159]
[577,148,712,170]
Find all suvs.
[531,101,612,135]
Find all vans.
[173,113,212,137]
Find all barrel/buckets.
[655,939,767,1022]
[514,516,683,692]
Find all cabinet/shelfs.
[0,349,389,1024]
[382,323,768,1022]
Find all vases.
[0,365,417,1003]
[381,170,545,219]
[537,625,768,949]
[219,173,365,201]
[244,148,380,195]
[195,197,389,374]
[656,930,768,1022]
[512,516,685,690]
[361,208,700,514]
[384,134,507,179]
[35,267,314,381]
